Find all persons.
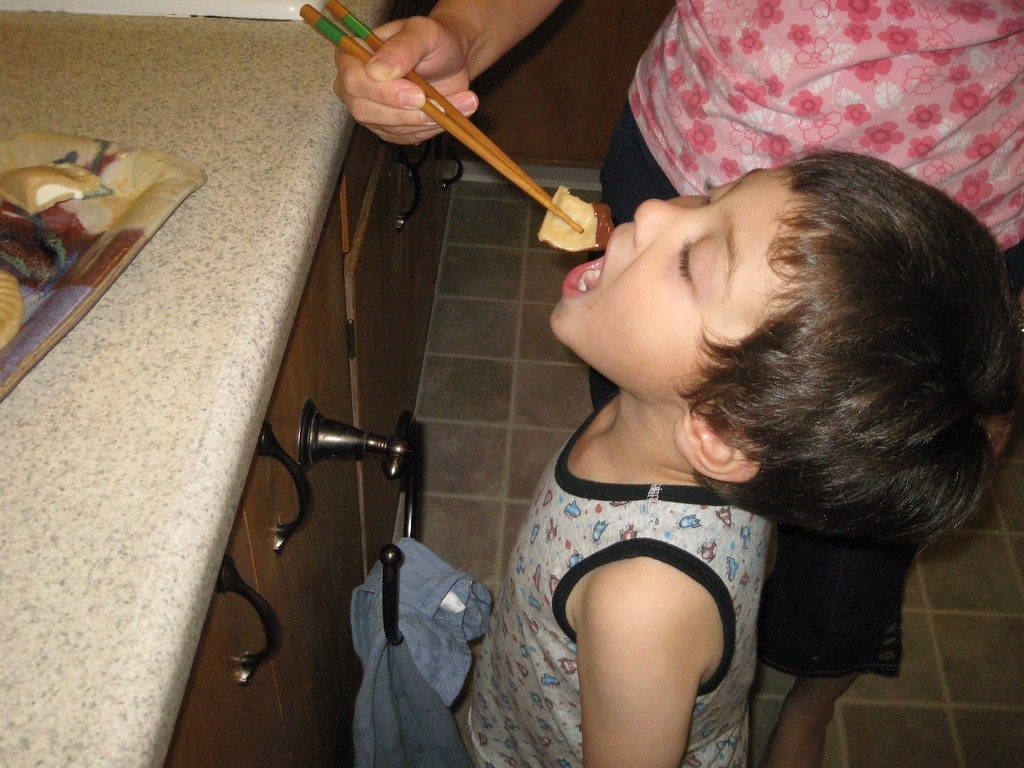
[334,0,1024,768]
[468,150,1024,768]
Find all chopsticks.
[300,1,585,236]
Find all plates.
[0,129,208,405]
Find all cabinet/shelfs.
[336,118,464,574]
[164,178,366,767]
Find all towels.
[351,538,494,767]
